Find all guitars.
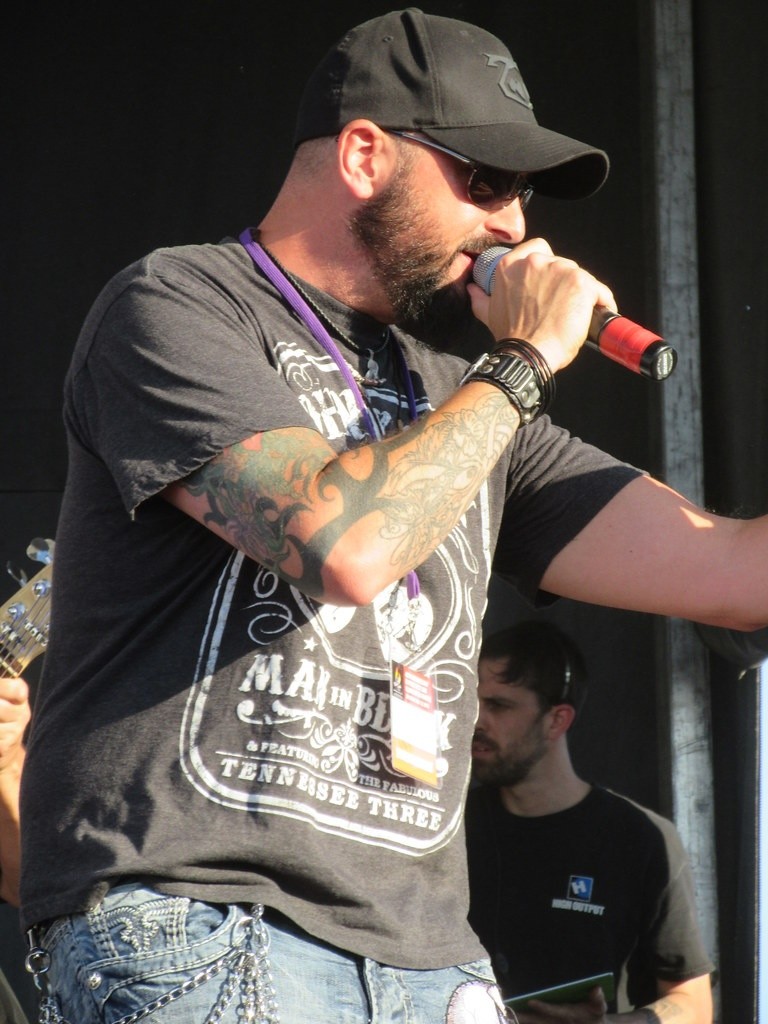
[1,536,57,684]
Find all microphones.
[472,247,679,382]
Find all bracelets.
[455,338,557,424]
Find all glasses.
[380,125,536,215]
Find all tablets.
[502,973,616,1021]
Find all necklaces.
[248,231,404,439]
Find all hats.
[296,6,610,201]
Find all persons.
[463,618,722,1024]
[0,11,768,1024]
[0,675,41,1024]
